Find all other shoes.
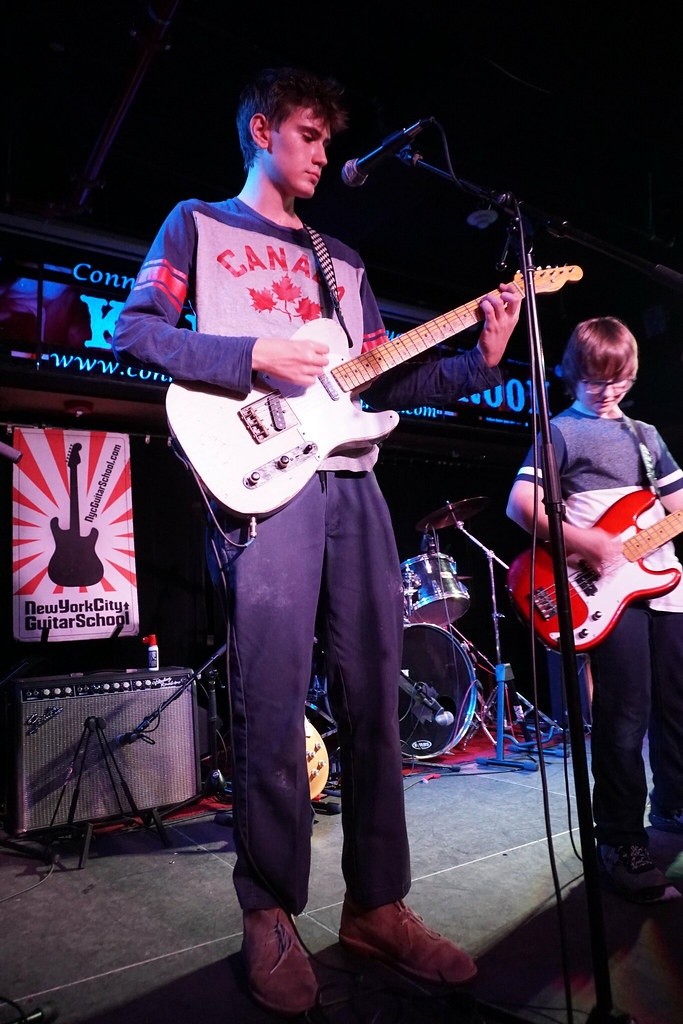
[648,798,683,833]
[597,842,667,897]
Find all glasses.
[576,375,637,394]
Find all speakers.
[9,667,202,837]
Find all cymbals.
[413,495,490,532]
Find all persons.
[115,72,522,1015]
[508,316,683,889]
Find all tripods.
[131,643,342,832]
[451,511,569,752]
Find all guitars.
[164,262,584,522]
[505,488,683,657]
[304,714,330,800]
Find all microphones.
[342,117,434,188]
[435,711,454,727]
[115,731,142,747]
[421,529,433,553]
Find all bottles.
[142,634,159,671]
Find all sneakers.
[338,892,478,982]
[242,906,318,1012]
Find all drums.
[322,622,478,760]
[400,551,472,627]
